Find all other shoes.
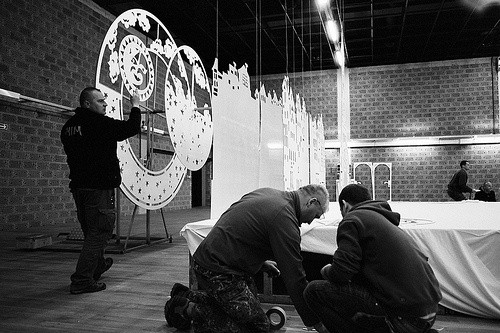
[164,295,188,329]
[95,256,113,278]
[170,282,192,299]
[70,281,106,295]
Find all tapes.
[266,306,286,330]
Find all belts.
[406,316,437,330]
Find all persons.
[474,181,497,202]
[164,182,330,333]
[59,86,143,296]
[301,183,445,333]
[446,160,478,202]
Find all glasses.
[316,199,326,220]
